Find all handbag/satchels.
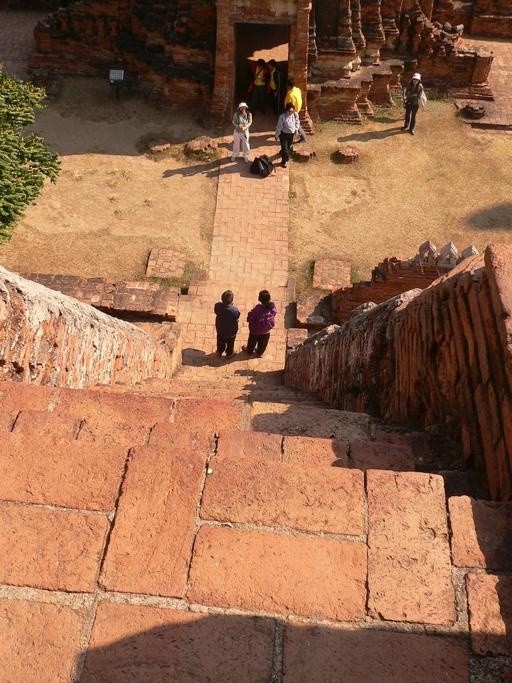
[418,90,428,111]
[250,154,277,177]
[246,79,254,92]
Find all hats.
[236,101,249,109]
[412,72,422,80]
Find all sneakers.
[230,156,249,164]
[401,126,416,136]
[278,150,288,168]
[215,344,264,360]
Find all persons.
[274,102,307,167]
[242,289,278,356]
[403,72,427,135]
[248,58,269,113]
[231,101,253,163]
[283,79,308,143]
[214,289,241,359]
[266,58,284,114]
